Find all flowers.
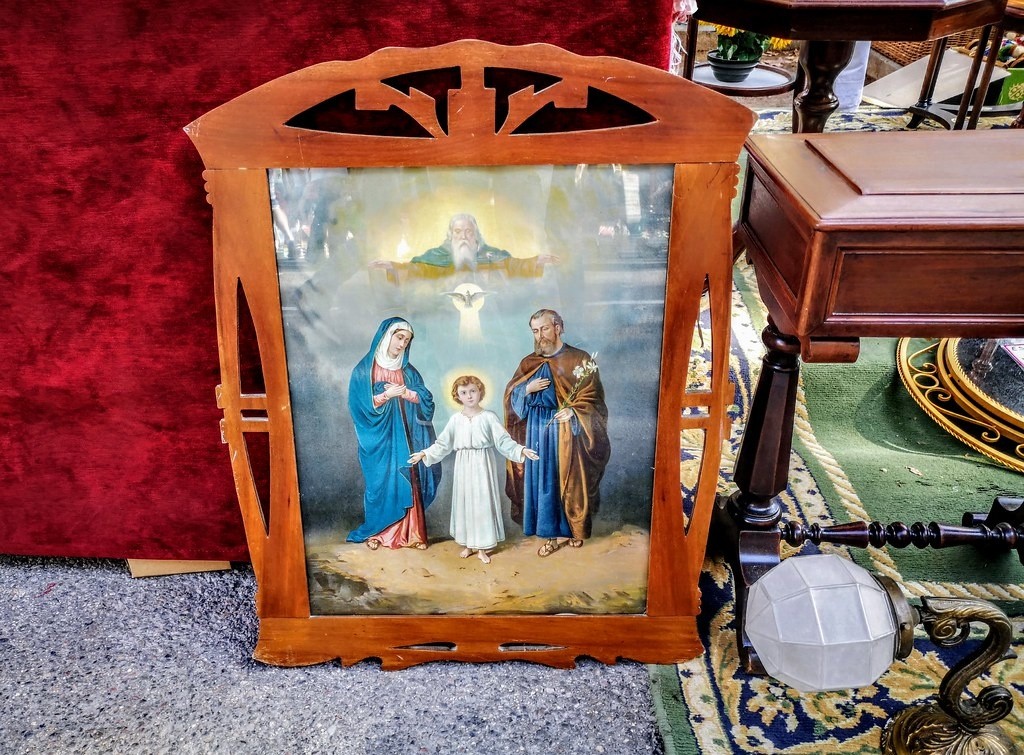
[698,19,792,61]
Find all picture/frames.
[186,42,757,680]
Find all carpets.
[635,103,1024,755]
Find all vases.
[706,50,760,83]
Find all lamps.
[747,554,1015,755]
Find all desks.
[679,0,1008,132]
[703,125,1024,680]
